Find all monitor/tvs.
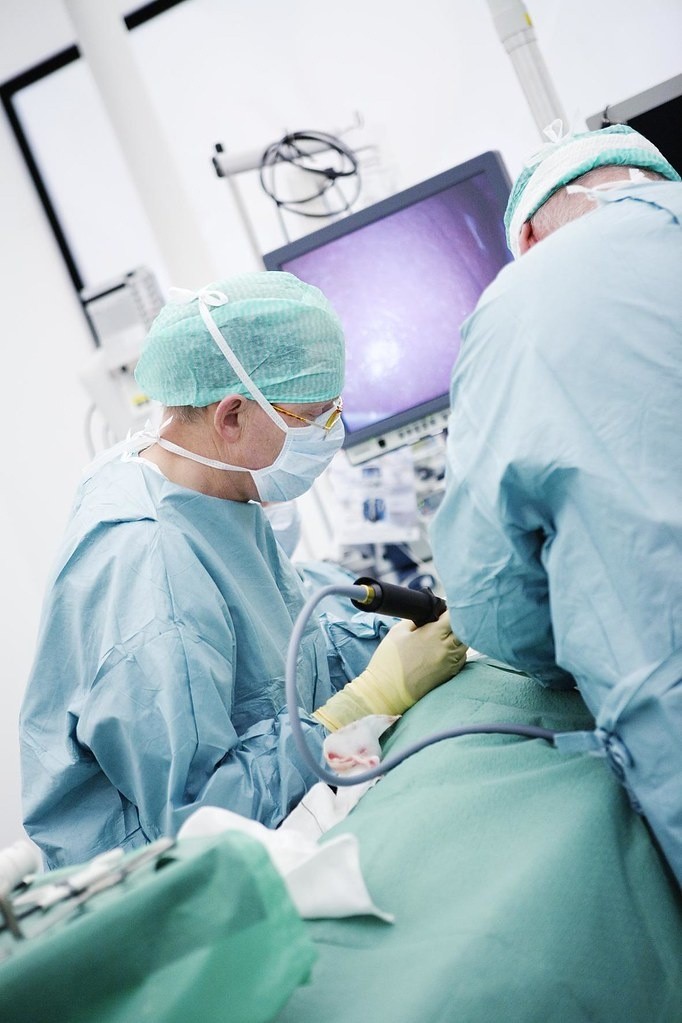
[262,147,512,452]
[586,72,682,178]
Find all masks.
[231,389,345,505]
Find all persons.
[430,125,682,886]
[260,499,361,618]
[16,272,466,875]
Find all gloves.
[309,606,471,735]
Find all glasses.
[238,395,343,431]
